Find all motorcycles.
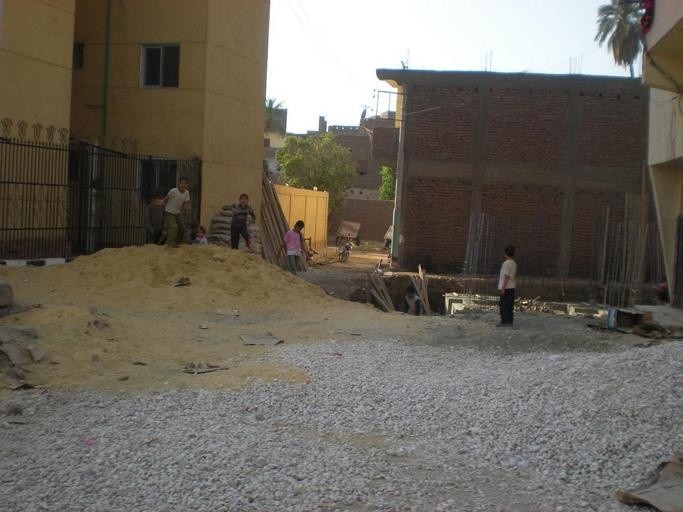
[336,233,354,263]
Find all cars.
[657,271,669,302]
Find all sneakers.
[494,322,510,329]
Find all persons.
[222,193,257,253]
[276,221,307,275]
[657,274,667,298]
[145,176,208,245]
[403,285,421,316]
[496,245,518,328]
